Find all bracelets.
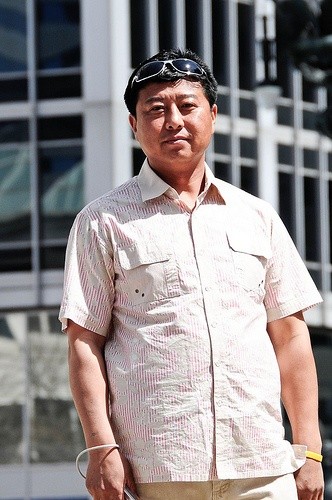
[305,451,323,462]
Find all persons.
[59,48,325,500]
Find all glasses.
[129,58,213,88]
